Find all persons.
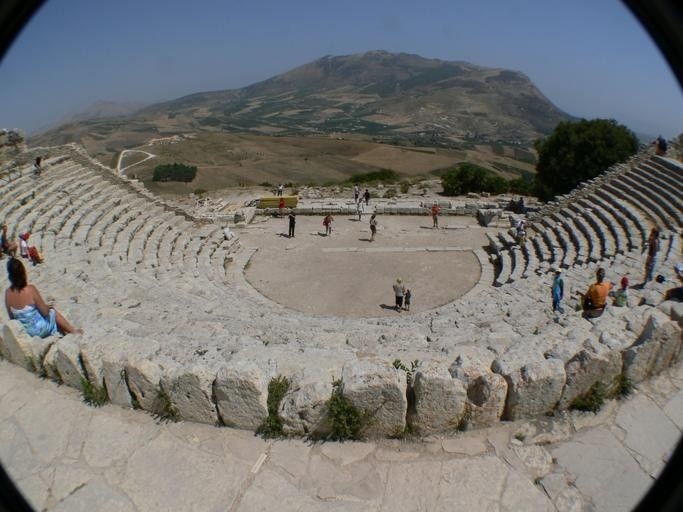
[273,183,540,247]
[664,263,682,302]
[611,277,629,308]
[33,155,43,178]
[3,258,84,340]
[402,288,410,313]
[576,268,613,317]
[547,267,563,312]
[637,226,660,288]
[391,277,405,313]
[0,223,45,265]
[648,134,667,151]
